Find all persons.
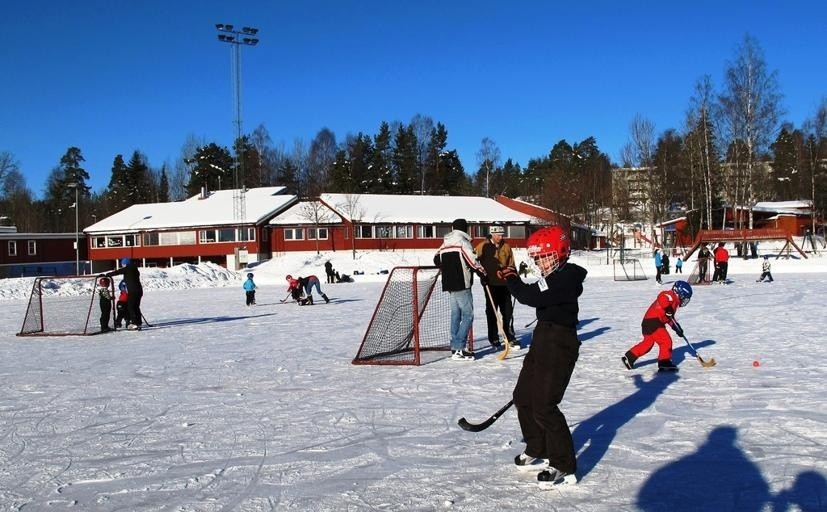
[325,257,333,284]
[433,219,487,360]
[243,273,255,306]
[652,241,776,282]
[98,278,112,331]
[98,257,144,330]
[285,275,305,299]
[113,281,133,328]
[517,261,527,278]
[472,225,520,347]
[502,227,587,482]
[623,281,692,370]
[293,275,329,305]
[332,269,336,283]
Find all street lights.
[91,214,96,224]
[66,182,82,278]
[215,22,259,250]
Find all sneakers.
[247,302,256,306]
[128,324,142,331]
[537,466,577,490]
[509,340,521,350]
[656,280,663,285]
[101,327,115,332]
[696,280,726,285]
[452,350,475,361]
[115,323,122,328]
[624,351,635,370]
[491,341,502,350]
[514,451,549,469]
[658,360,678,370]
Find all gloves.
[105,273,112,278]
[665,309,673,320]
[497,266,518,282]
[481,276,490,286]
[676,328,684,337]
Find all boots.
[308,295,313,305]
[322,293,329,304]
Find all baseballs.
[753,361,759,367]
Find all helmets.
[671,280,693,308]
[100,278,110,287]
[525,226,571,278]
[247,273,253,278]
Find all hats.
[121,258,131,265]
[452,219,467,233]
[489,221,506,235]
